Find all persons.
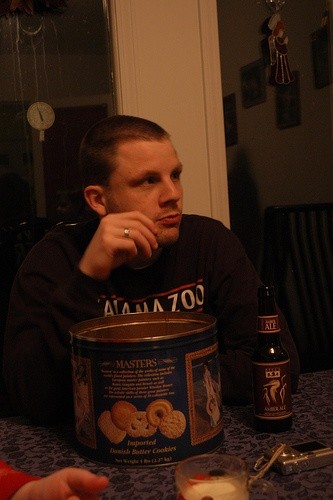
[0,455,111,500]
[0,154,34,302]
[2,116,300,427]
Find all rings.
[124,226,128,239]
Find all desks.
[0,367,333,500]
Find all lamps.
[21,101,55,142]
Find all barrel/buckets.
[68,311,225,466]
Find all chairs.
[262,204,332,371]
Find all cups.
[175,453,277,500]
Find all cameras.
[263,440,333,475]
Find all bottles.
[250,286,294,432]
